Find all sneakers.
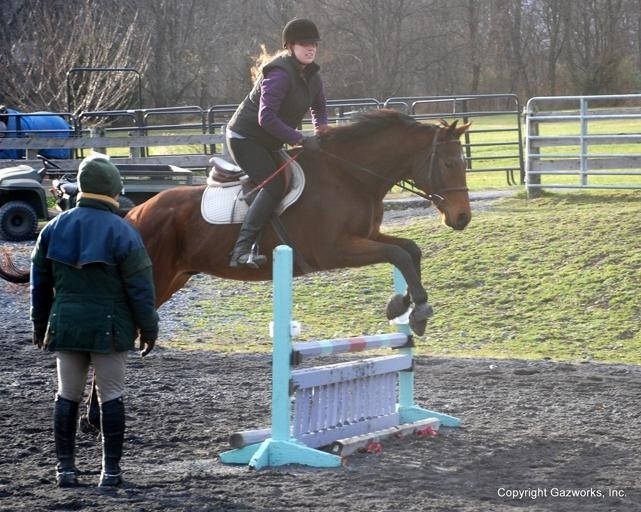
[55,463,77,485]
[98,467,123,489]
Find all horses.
[0,107,473,337]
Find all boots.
[229,187,267,267]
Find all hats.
[79,155,123,197]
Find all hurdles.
[218,245,463,470]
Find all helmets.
[283,17,320,44]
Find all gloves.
[31,320,49,347]
[303,134,320,151]
[137,328,158,357]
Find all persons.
[226,19,329,267]
[31,158,158,487]
